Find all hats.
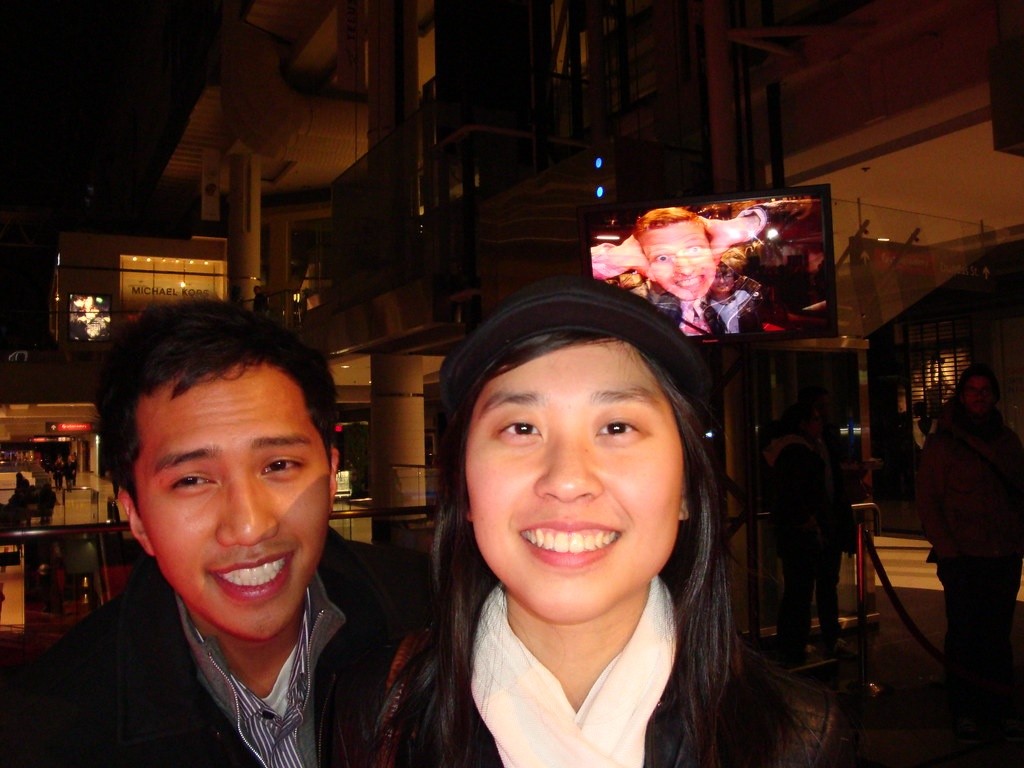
[438,276,725,465]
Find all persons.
[761,387,859,665]
[915,361,1024,745]
[321,274,866,768]
[4,452,78,530]
[0,292,428,768]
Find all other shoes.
[823,638,858,662]
[780,642,806,670]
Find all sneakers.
[952,714,981,745]
[998,716,1024,742]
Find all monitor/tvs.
[577,183,837,347]
